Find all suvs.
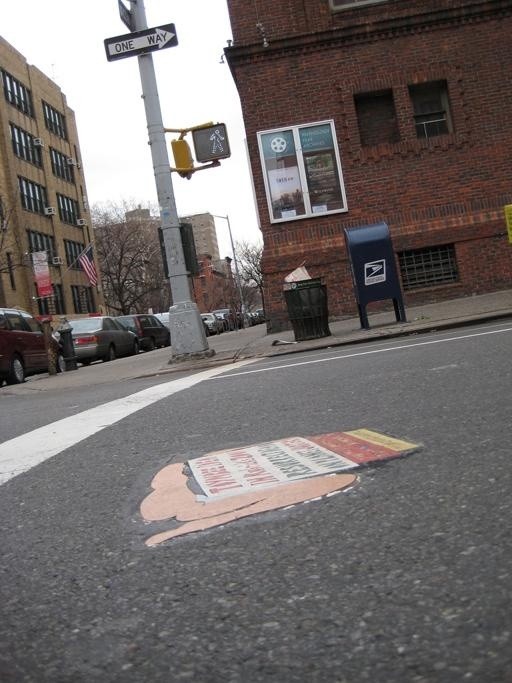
[0,306,65,385]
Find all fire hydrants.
[56,315,79,371]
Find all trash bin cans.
[282,275,331,342]
[343,221,407,330]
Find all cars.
[117,307,265,353]
[53,316,140,365]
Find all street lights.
[210,214,245,312]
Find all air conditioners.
[68,158,77,165]
[35,139,45,148]
[45,207,58,216]
[54,257,65,265]
[78,219,88,226]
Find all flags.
[79,244,98,287]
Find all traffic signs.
[103,22,179,64]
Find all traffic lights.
[171,139,194,178]
[193,122,231,164]
